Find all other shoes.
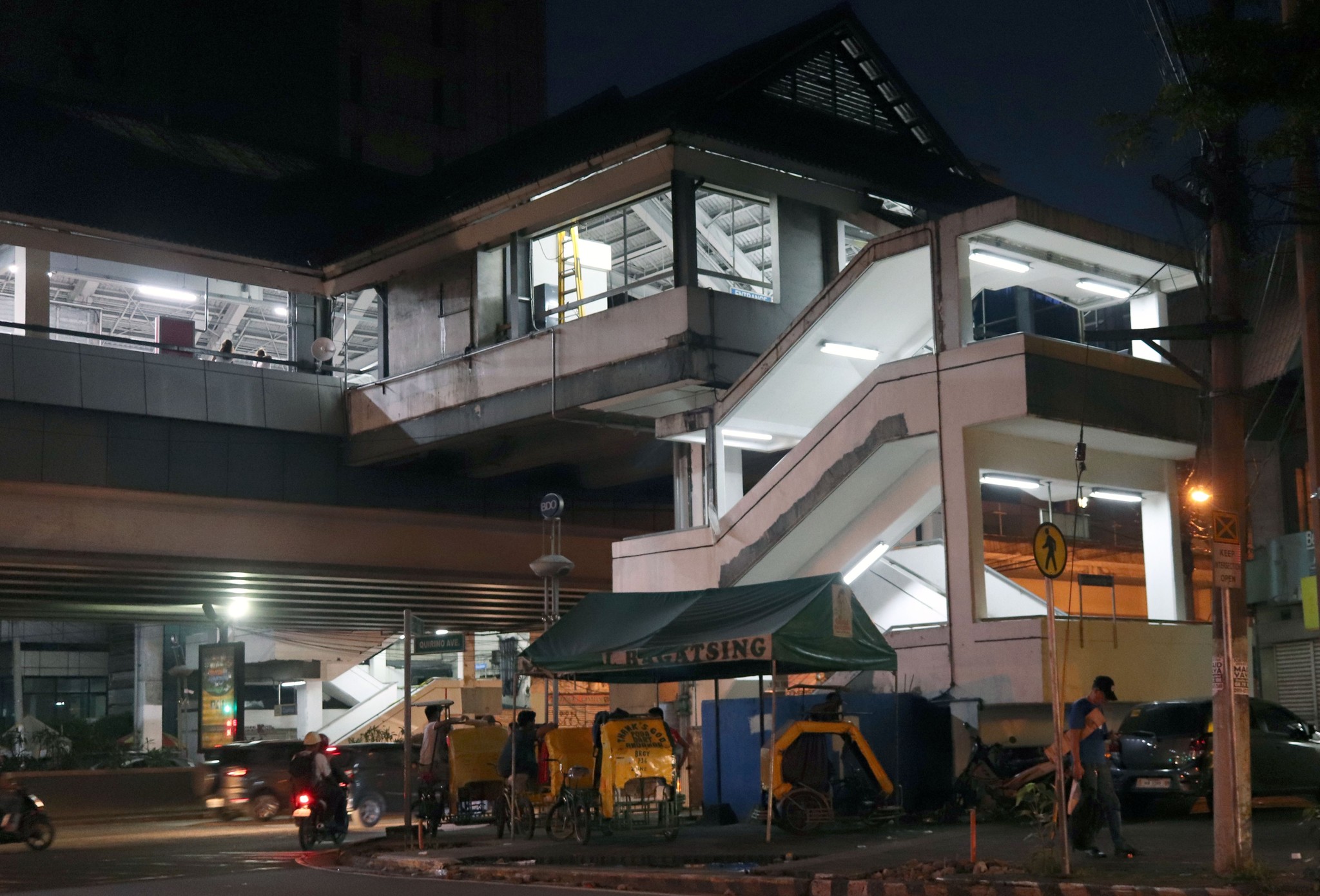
[1114,848,1141,856]
[1085,847,1106,858]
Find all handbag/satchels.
[1065,776,1107,851]
[420,770,437,785]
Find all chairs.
[549,729,599,786]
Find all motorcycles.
[292,764,356,849]
[0,780,55,853]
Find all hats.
[1094,676,1117,701]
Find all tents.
[511,572,899,842]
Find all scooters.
[943,721,1070,828]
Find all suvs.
[199,739,312,822]
[326,744,433,828]
[1107,700,1320,815]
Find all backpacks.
[288,752,322,793]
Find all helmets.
[319,734,329,746]
[303,732,321,745]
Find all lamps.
[1074,277,1130,299]
[311,337,336,374]
[968,248,1031,274]
[811,340,881,360]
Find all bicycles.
[488,765,535,841]
[409,763,442,837]
[543,757,592,845]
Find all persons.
[290,731,354,834]
[211,339,233,363]
[419,704,559,793]
[648,708,689,777]
[1068,675,1138,857]
[252,350,272,369]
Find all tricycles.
[758,684,907,838]
[522,726,595,842]
[441,724,510,838]
[588,716,684,844]
